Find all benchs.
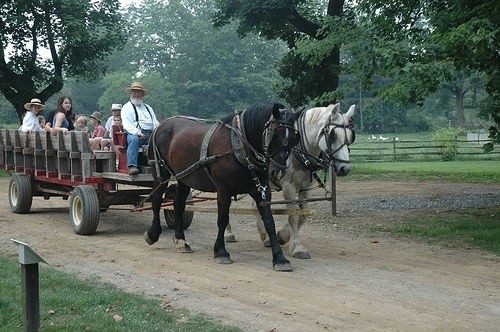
[0,129,116,185]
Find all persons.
[77,115,92,138]
[111,117,127,146]
[45,99,75,132]
[120,83,161,175]
[88,111,106,150]
[20,98,45,130]
[53,97,72,132]
[103,104,123,150]
[37,115,45,129]
[71,113,76,123]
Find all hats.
[24,98,46,110]
[111,104,122,110]
[89,111,102,123]
[124,82,150,96]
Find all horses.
[223,102,356,259]
[143,103,306,272]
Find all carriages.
[0,103,356,271]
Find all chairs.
[112,125,129,174]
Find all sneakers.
[129,166,140,176]
